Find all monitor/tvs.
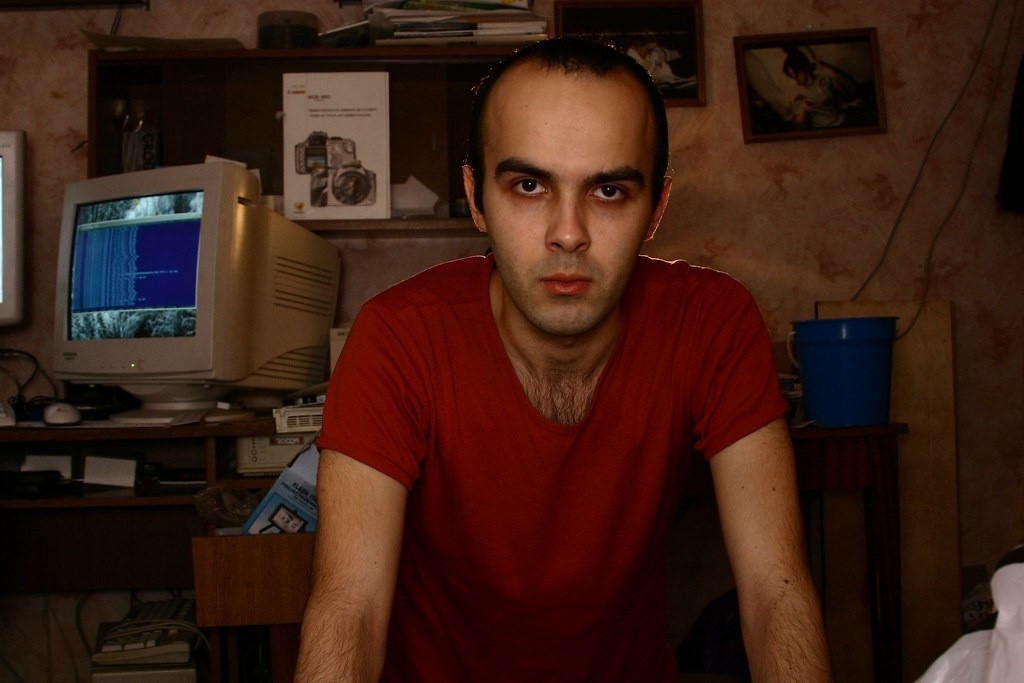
[51,160,340,424]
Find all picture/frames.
[554,0,707,108]
[735,26,888,144]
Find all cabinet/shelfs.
[83,39,556,233]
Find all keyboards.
[92,598,202,666]
[0,401,16,426]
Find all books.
[316,0,548,41]
[283,72,389,220]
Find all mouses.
[43,402,81,425]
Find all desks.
[795,424,907,682]
[0,421,280,683]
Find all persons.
[626,28,698,97]
[294,34,829,682]
[783,45,879,130]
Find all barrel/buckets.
[784,315,899,429]
